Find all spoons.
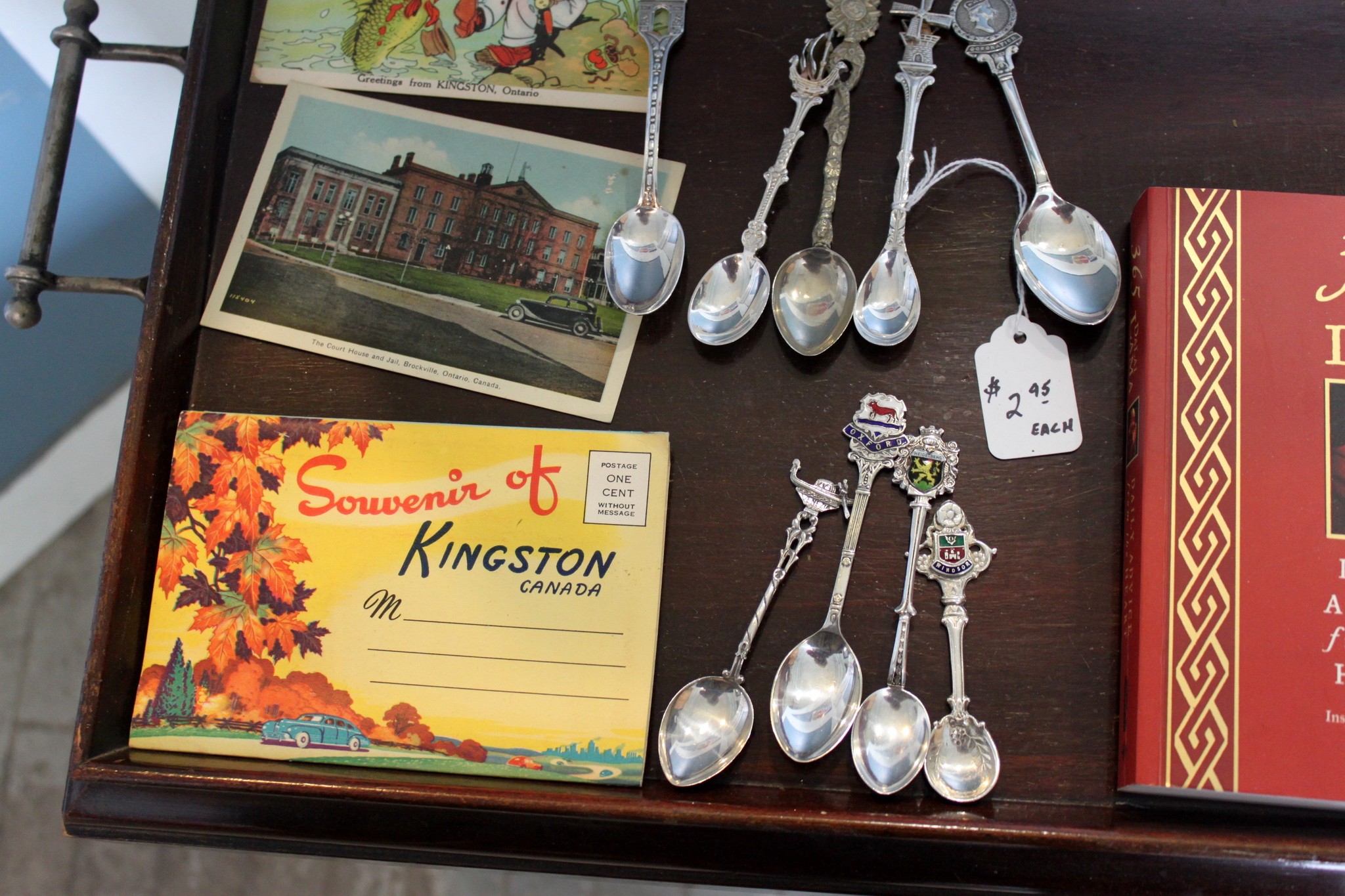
[686,30,840,343]
[658,458,855,788]
[950,1,1121,325]
[901,500,1000,803]
[851,425,960,795]
[603,0,686,316]
[769,391,911,763]
[853,0,955,346]
[770,1,883,357]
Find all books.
[1117,187,1345,812]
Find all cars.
[532,281,554,294]
[505,293,604,339]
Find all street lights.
[327,210,355,268]
[440,244,452,272]
